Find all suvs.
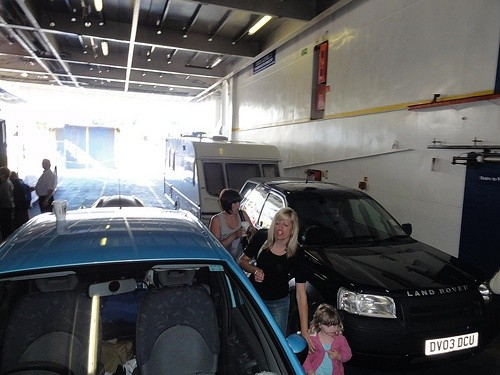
[237,177,498,368]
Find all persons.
[301,303,353,375]
[34,158,56,212]
[208,187,249,259]
[237,207,316,356]
[0,166,38,242]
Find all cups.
[241,221,251,237]
[51,199,68,219]
[56,221,66,236]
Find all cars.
[0,206,309,375]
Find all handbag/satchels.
[236,239,268,278]
[238,210,258,250]
